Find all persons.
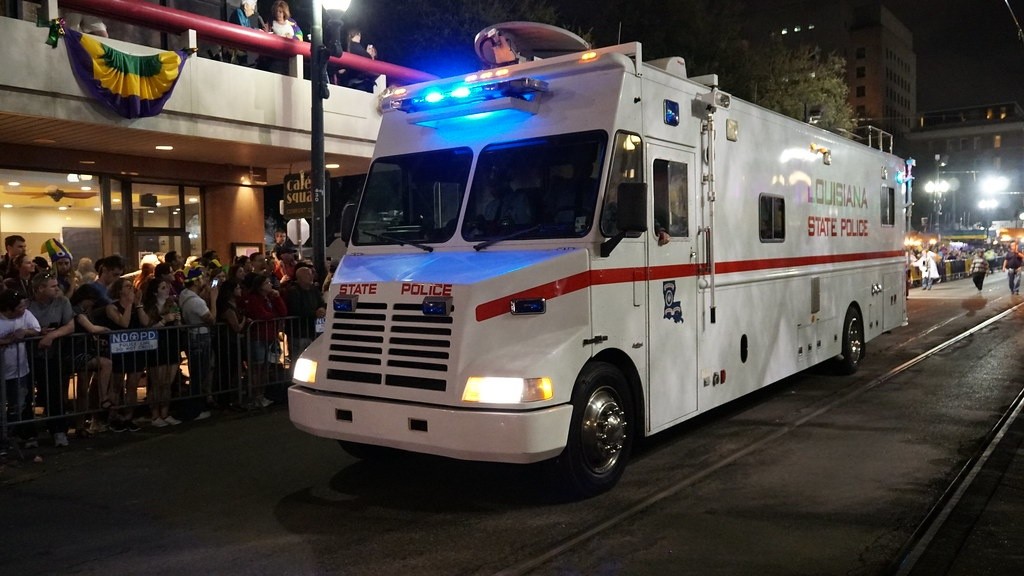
[232,0,267,70]
[908,241,1022,285]
[910,249,942,290]
[0,231,324,475]
[269,0,304,74]
[322,259,342,304]
[62,10,109,38]
[1002,241,1024,295]
[478,171,543,225]
[618,184,670,245]
[967,250,989,292]
[345,25,382,94]
[187,213,199,238]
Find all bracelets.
[135,304,143,309]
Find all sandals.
[99,398,121,410]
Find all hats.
[183,264,204,283]
[276,247,297,255]
[295,262,314,272]
[185,255,202,265]
[211,259,222,267]
[45,237,73,263]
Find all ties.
[491,200,504,222]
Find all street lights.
[310,0,353,290]
[924,179,950,234]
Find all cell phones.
[367,45,373,55]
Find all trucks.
[288,20,916,491]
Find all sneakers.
[164,416,182,425]
[54,433,69,447]
[25,438,38,448]
[109,423,127,432]
[90,422,108,434]
[124,421,141,432]
[151,418,168,427]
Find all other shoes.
[256,397,273,406]
